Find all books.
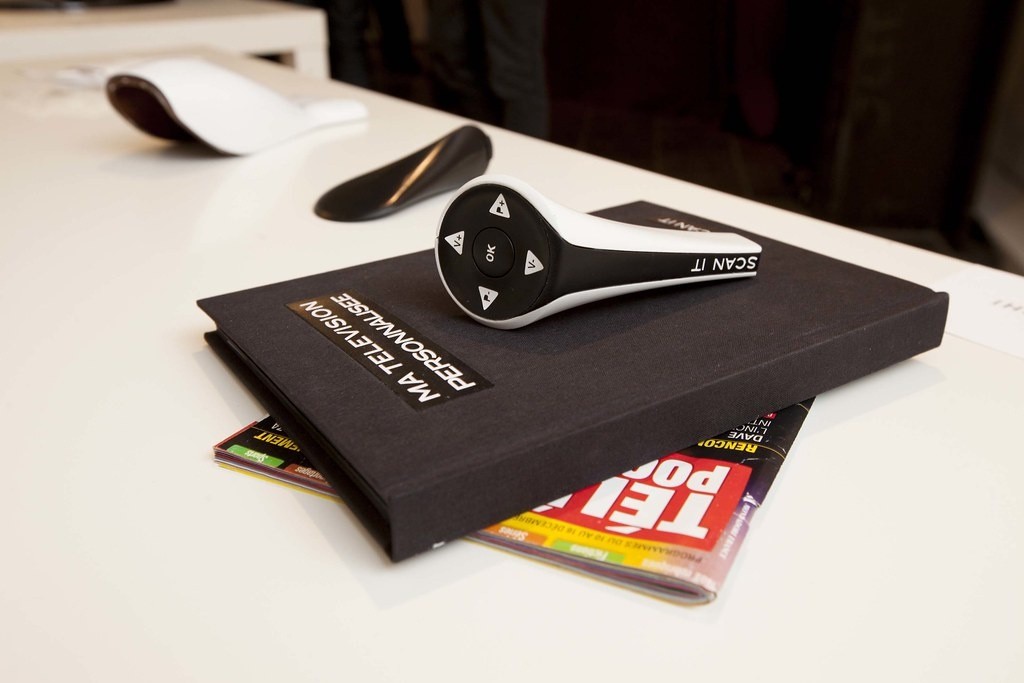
[189,199,948,565]
[213,395,816,606]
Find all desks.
[2,47,1024,652]
[1,0,329,79]
